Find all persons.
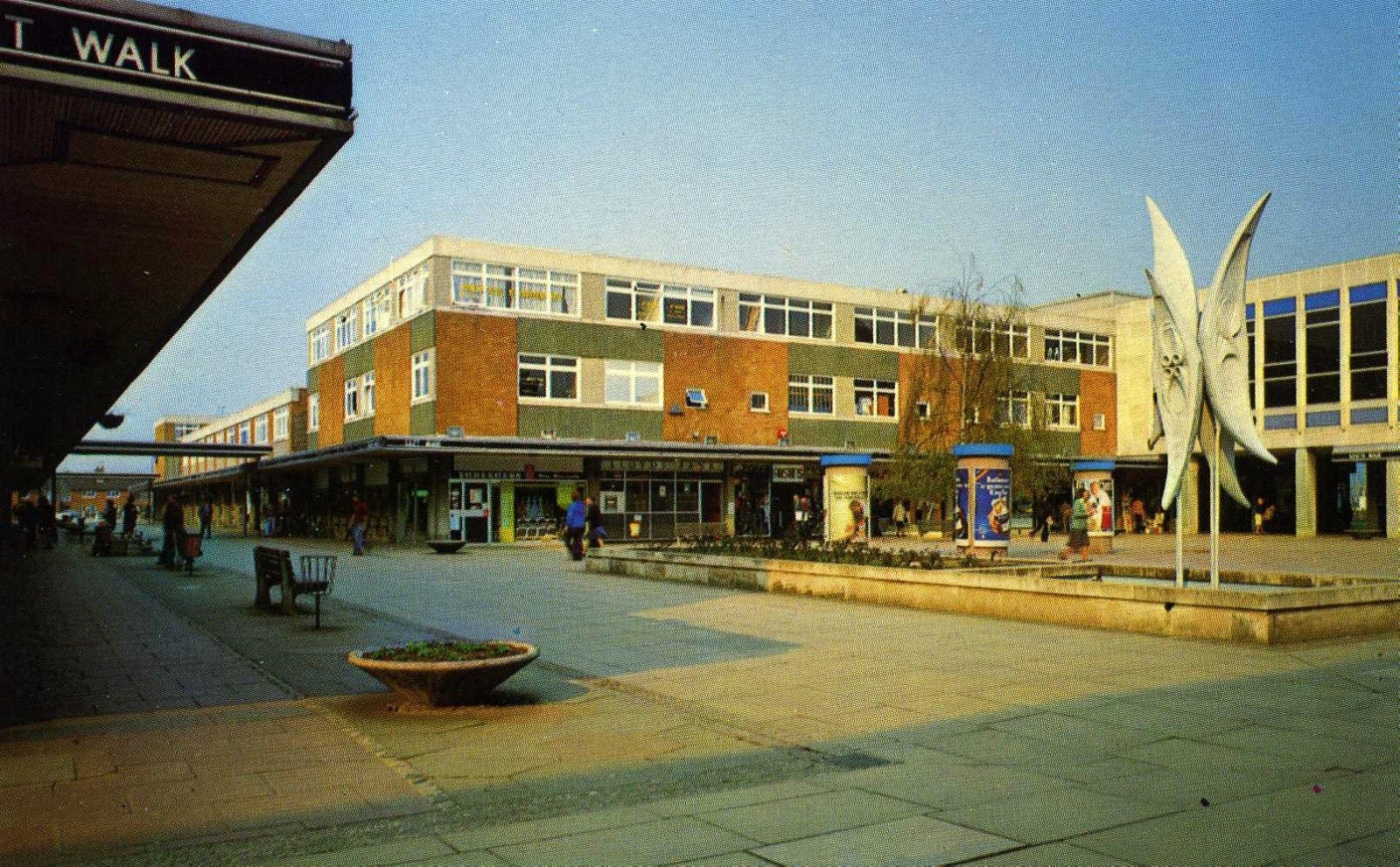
[892,499,908,538]
[1117,487,1276,539]
[1028,495,1051,541]
[348,494,368,555]
[17,490,212,570]
[1059,489,1094,560]
[583,495,608,551]
[562,491,589,562]
[1085,481,1111,529]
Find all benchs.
[252,544,330,619]
[917,519,956,540]
